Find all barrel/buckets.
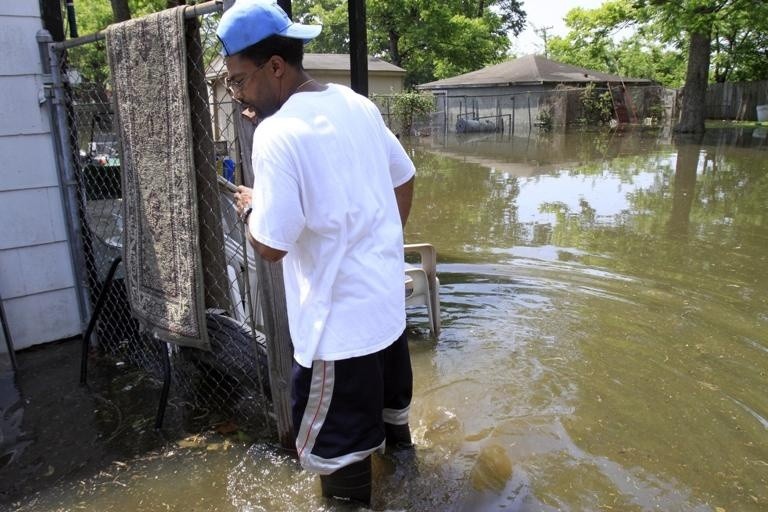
[756,104,768,122]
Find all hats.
[216,1,323,58]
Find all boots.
[317,453,374,512]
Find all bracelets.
[240,206,253,225]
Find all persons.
[216,0,423,511]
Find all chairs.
[81,162,266,351]
[405,240,441,337]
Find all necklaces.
[293,78,316,94]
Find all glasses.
[226,60,268,94]
